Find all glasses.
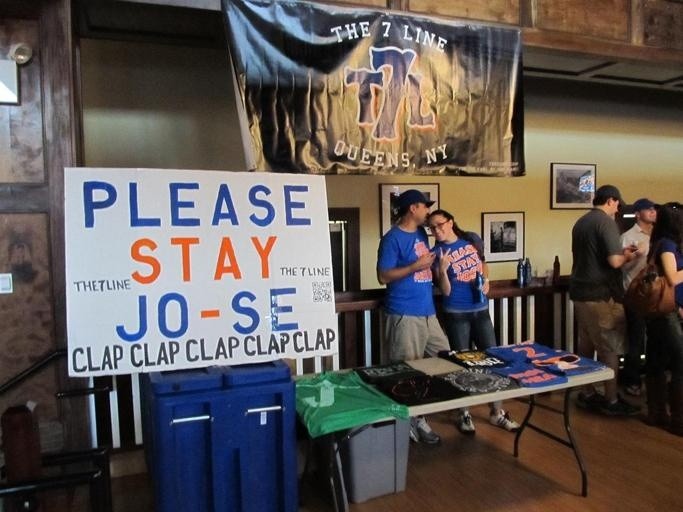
[427,220,449,231]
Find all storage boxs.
[341,417,411,504]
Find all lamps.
[0,44,33,103]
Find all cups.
[628,240,638,253]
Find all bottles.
[516,258,524,286]
[553,256,560,282]
[524,258,531,284]
[475,270,486,303]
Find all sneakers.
[457,415,475,435]
[577,386,642,415]
[409,415,440,444]
[489,409,521,432]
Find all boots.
[645,376,682,436]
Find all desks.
[295,336,614,510]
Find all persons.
[568,185,642,417]
[649,202,682,436]
[377,189,452,445]
[426,209,522,433]
[619,197,658,396]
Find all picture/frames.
[378,181,440,238]
[481,211,525,264]
[549,162,596,211]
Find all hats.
[398,189,435,207]
[632,199,659,212]
[597,185,625,206]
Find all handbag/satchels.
[624,265,676,315]
